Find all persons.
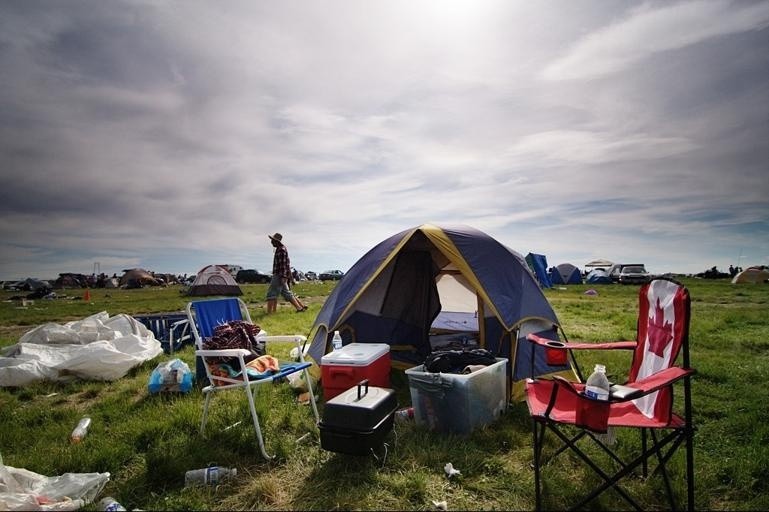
[272,256,309,312]
[86,271,118,289]
[263,232,306,317]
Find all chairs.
[185,297,321,460]
[523,274,700,512]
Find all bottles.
[99,495,129,512]
[70,417,91,446]
[583,363,609,402]
[330,330,343,350]
[185,467,238,485]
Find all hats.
[268,233,284,246]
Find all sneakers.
[297,305,308,313]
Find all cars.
[4,281,25,290]
[618,266,651,284]
[236,269,272,284]
[319,270,345,280]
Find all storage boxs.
[321,342,391,401]
[404,358,509,436]
[318,385,399,455]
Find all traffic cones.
[84,288,91,300]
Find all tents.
[282,222,591,413]
[181,264,246,298]
